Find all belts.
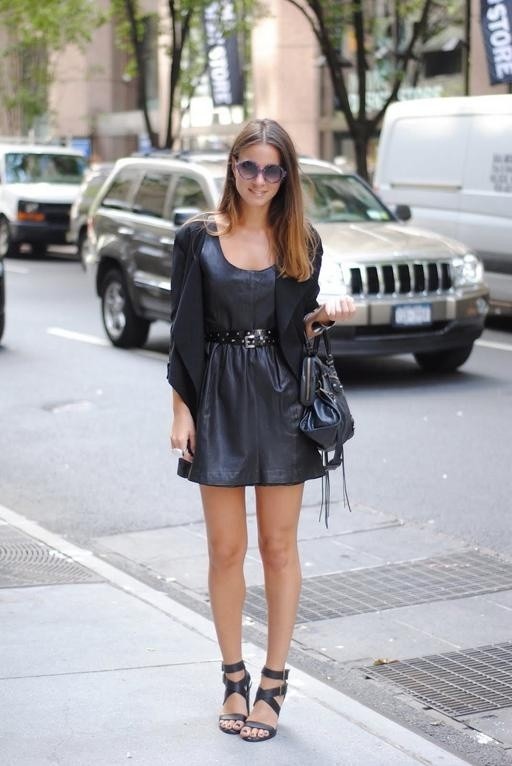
[207,327,275,349]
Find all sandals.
[219,661,252,735]
[239,666,289,743]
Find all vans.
[1,130,94,261]
[364,93,512,324]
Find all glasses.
[233,160,287,186]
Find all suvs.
[80,147,491,377]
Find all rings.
[171,449,188,457]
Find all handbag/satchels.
[299,352,355,454]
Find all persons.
[165,118,357,741]
